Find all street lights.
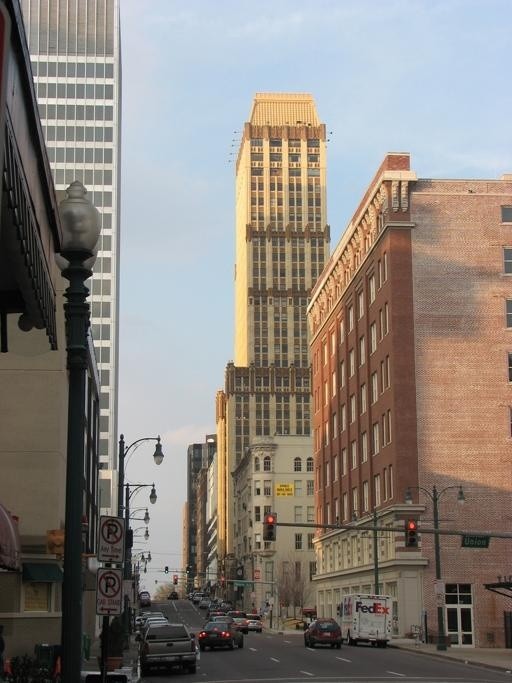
[404,482,465,651]
[48,179,103,683]
[350,505,400,595]
[118,434,164,647]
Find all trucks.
[335,594,392,649]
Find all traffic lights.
[264,511,278,543]
[165,566,169,574]
[173,574,178,587]
[185,567,190,574]
[405,517,418,548]
[220,575,225,585]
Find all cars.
[140,590,264,674]
[304,616,343,650]
